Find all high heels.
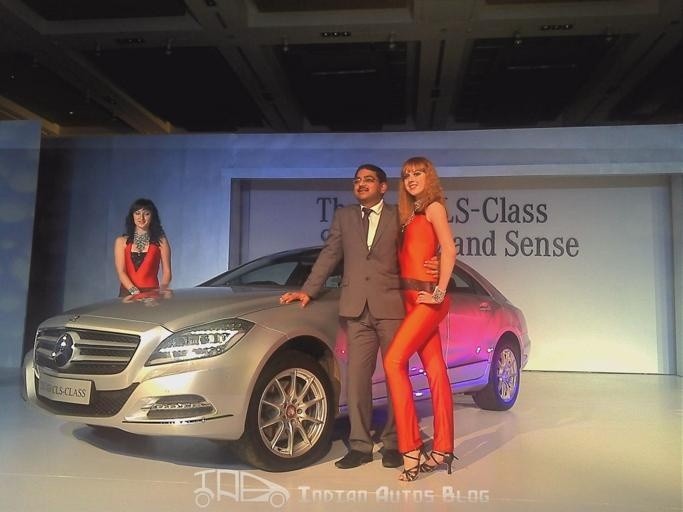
[420,451,454,474]
[399,443,430,482]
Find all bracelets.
[126,285,138,295]
[432,286,446,304]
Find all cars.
[19,244,531,473]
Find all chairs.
[290,269,323,290]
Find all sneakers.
[335,448,373,469]
[382,448,404,468]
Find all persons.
[278,162,443,471]
[111,196,172,301]
[379,155,460,485]
[121,287,175,309]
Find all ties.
[362,207,374,246]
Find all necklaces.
[130,231,154,256]
[400,196,428,231]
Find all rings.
[286,292,292,295]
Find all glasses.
[353,176,375,185]
